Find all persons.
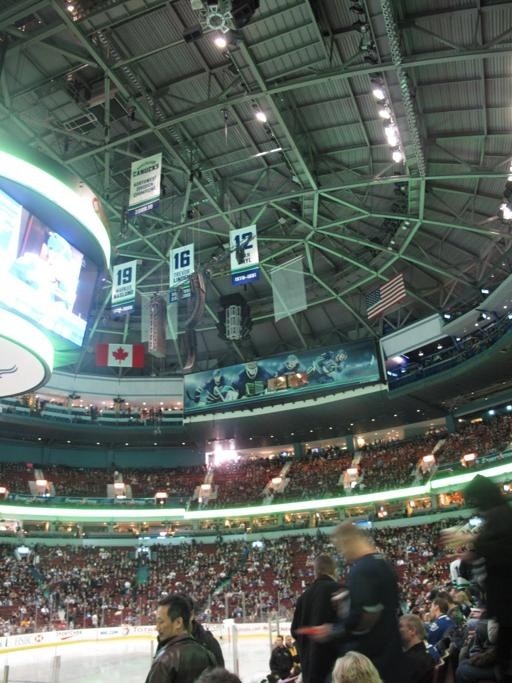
[275,354,307,390]
[1,458,269,637]
[144,594,219,683]
[227,362,276,401]
[270,415,512,682]
[195,666,243,683]
[156,593,227,669]
[307,347,349,387]
[192,368,233,405]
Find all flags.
[93,341,146,370]
[363,273,408,323]
[229,224,260,286]
[169,242,195,302]
[111,259,137,317]
